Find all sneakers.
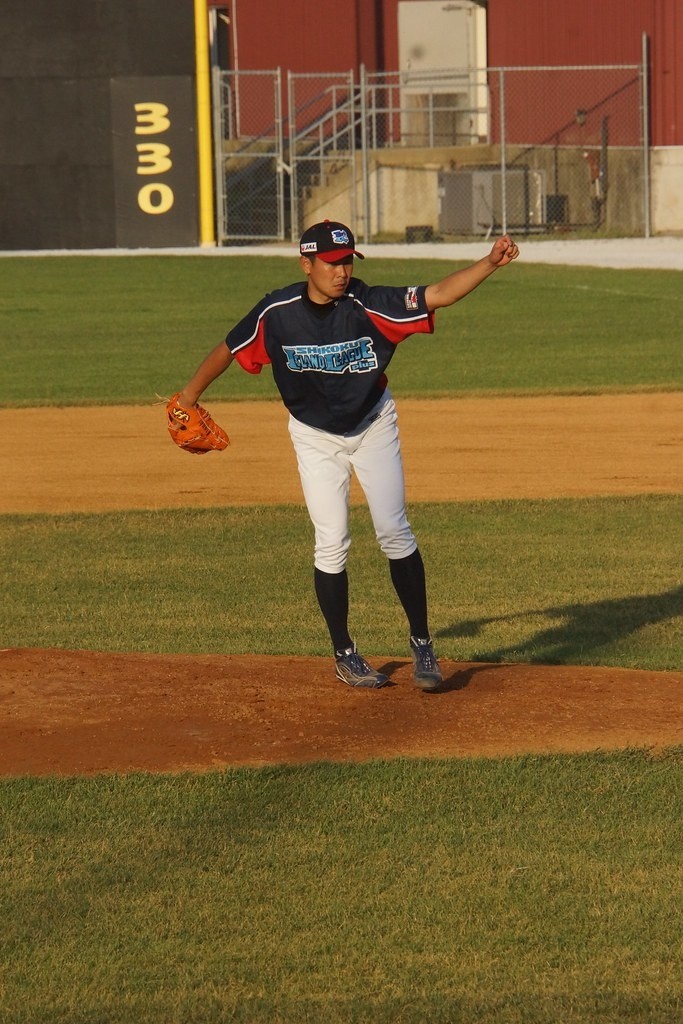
[334,642,388,688]
[410,636,443,690]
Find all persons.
[166,220,520,689]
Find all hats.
[300,220,365,263]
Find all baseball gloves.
[153,392,230,454]
